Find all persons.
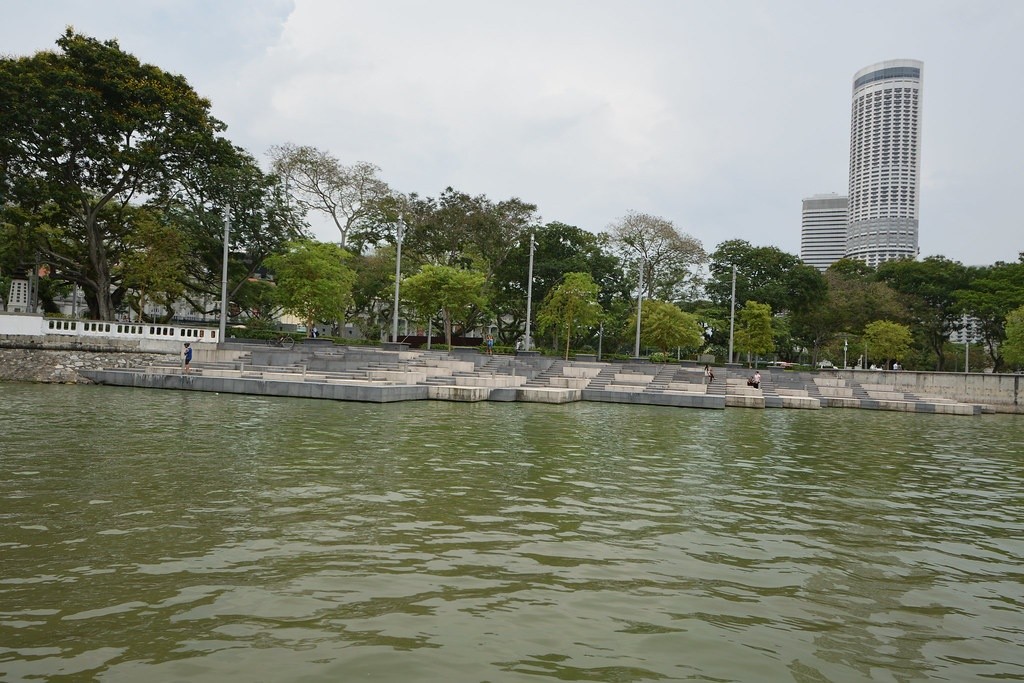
[893,361,898,370]
[704,364,717,384]
[486,335,493,355]
[516,338,525,355]
[311,324,319,338]
[184,343,192,369]
[748,371,762,389]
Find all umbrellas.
[232,325,247,331]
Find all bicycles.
[269,334,294,348]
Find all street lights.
[220,204,236,343]
[383,212,411,351]
[517,234,541,357]
